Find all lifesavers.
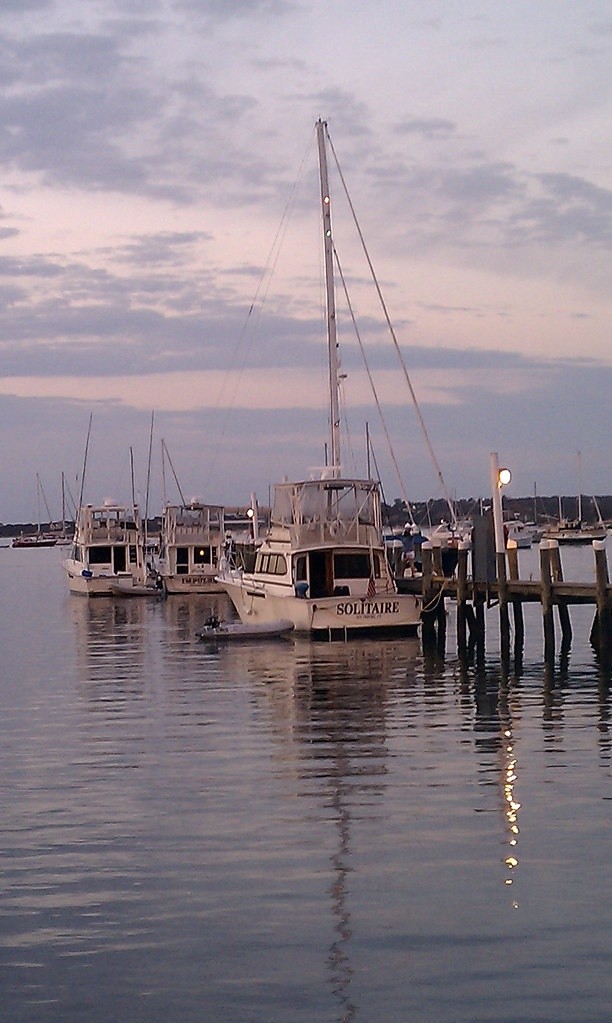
[330,520,346,538]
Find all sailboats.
[315,117,472,578]
[430,451,608,549]
[10,472,78,547]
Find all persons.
[223,534,236,571]
[402,522,416,579]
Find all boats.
[60,410,156,598]
[196,617,296,639]
[107,584,167,598]
[212,441,427,643]
[144,437,230,593]
